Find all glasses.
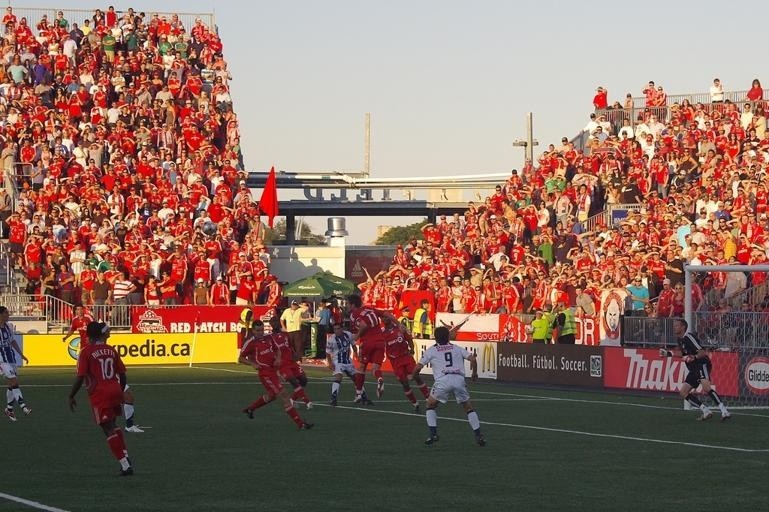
[0,8,261,284]
[376,84,769,293]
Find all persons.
[357,79,769,351]
[660,319,731,420]
[237,308,313,430]
[0,305,32,422]
[412,327,486,445]
[1,4,280,329]
[280,295,430,410]
[69,321,134,477]
[63,303,144,432]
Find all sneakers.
[125,425,144,433]
[289,397,298,408]
[120,458,134,476]
[413,403,420,412]
[353,392,371,406]
[377,378,385,400]
[329,398,337,406]
[4,408,17,422]
[23,407,32,417]
[241,406,254,419]
[424,434,439,444]
[297,423,315,432]
[305,402,313,411]
[720,414,732,422]
[696,411,714,421]
[477,437,487,446]
